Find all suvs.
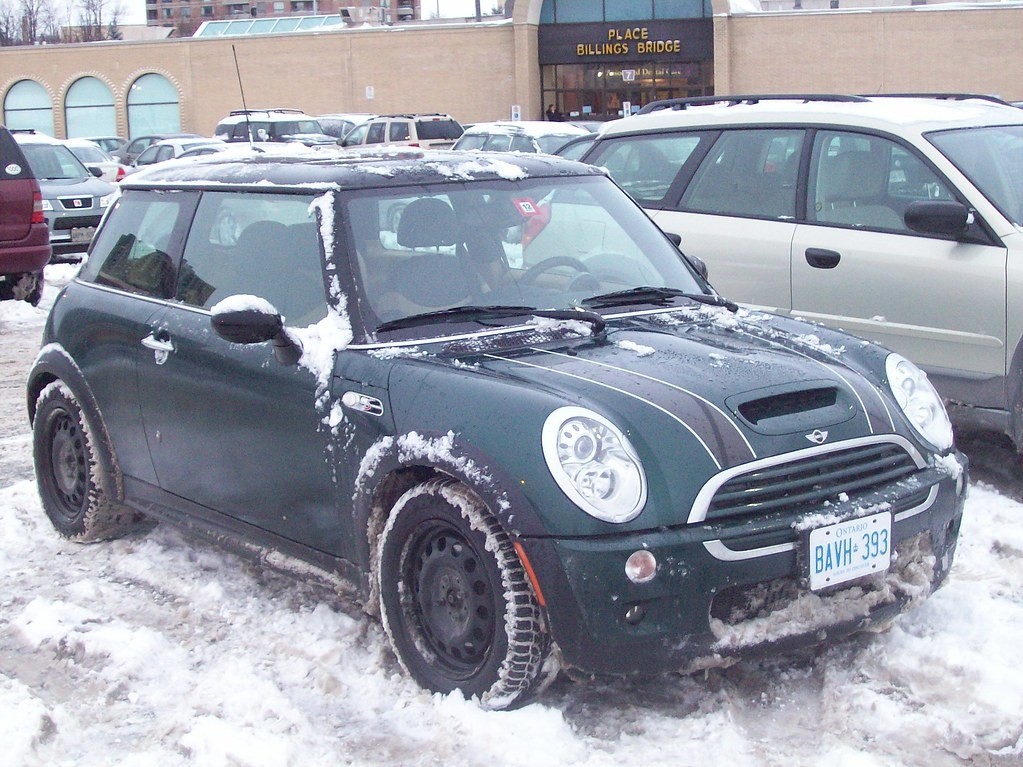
[303,113,399,146]
[6,128,120,254]
[214,108,341,151]
[450,121,632,170]
[336,113,465,150]
[522,94,1022,463]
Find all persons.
[546,103,561,122]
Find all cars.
[84,133,317,176]
[55,139,129,183]
[26,145,968,711]
[0,122,52,307]
[599,115,1023,185]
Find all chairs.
[688,151,907,229]
[226,197,473,326]
